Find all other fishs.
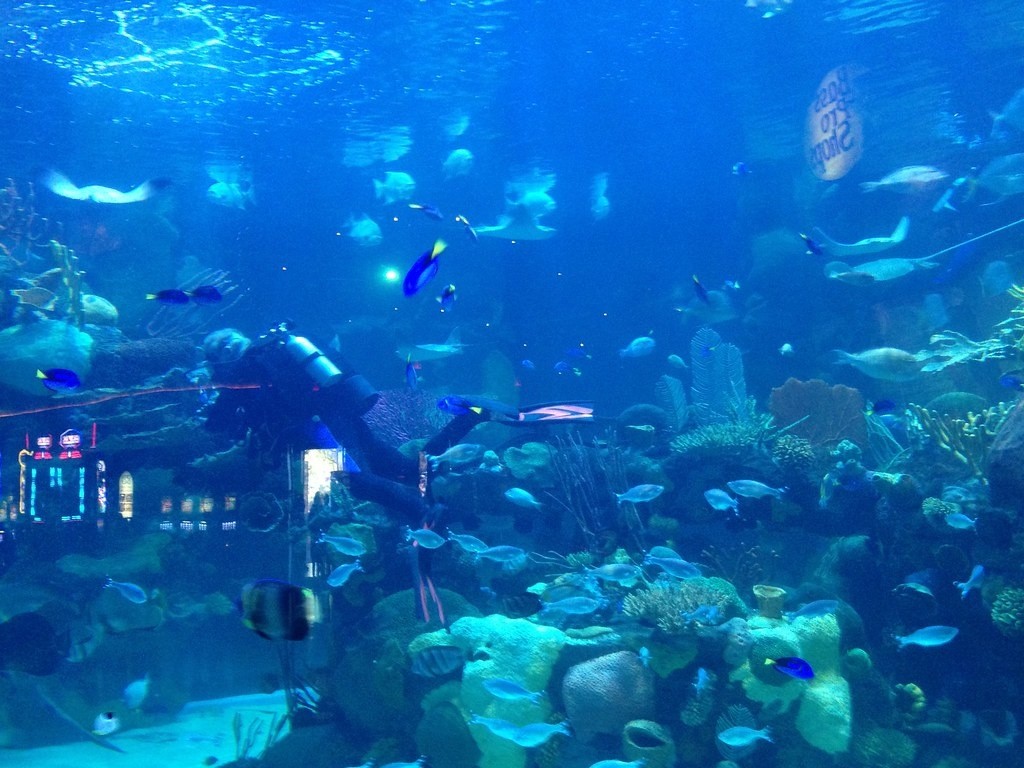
[23,85,1024,768]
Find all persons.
[170,328,597,630]
